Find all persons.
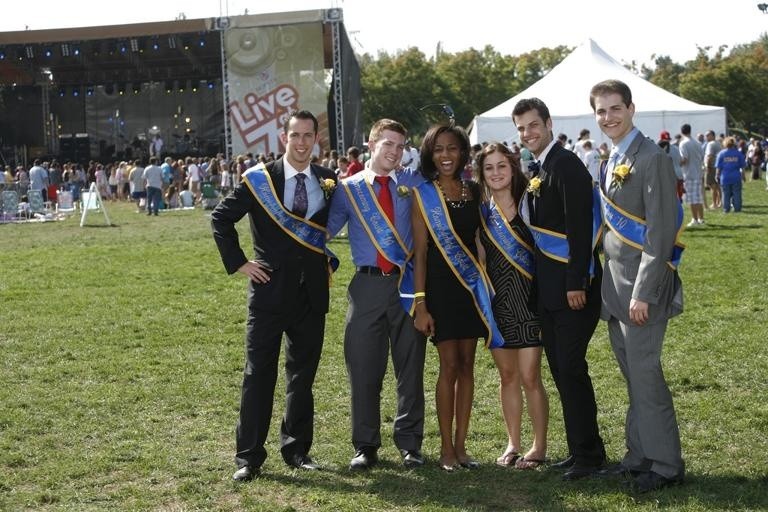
[473,143,550,469]
[657,124,768,226]
[412,126,480,473]
[400,139,419,170]
[311,139,370,178]
[210,110,339,479]
[512,97,605,479]
[463,141,530,183]
[324,118,424,470]
[587,81,685,494]
[557,129,608,181]
[2,133,282,216]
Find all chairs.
[0,191,29,223]
[26,189,52,219]
[80,189,100,214]
[55,190,77,218]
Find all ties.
[291,174,308,218]
[375,175,395,274]
[604,152,619,196]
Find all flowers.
[610,164,630,192]
[396,186,410,198]
[526,175,543,198]
[319,176,336,199]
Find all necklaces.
[435,175,468,210]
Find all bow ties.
[528,160,541,226]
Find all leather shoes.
[292,453,322,471]
[442,463,460,472]
[596,463,629,476]
[551,456,577,469]
[232,465,260,482]
[623,471,683,492]
[463,459,479,471]
[349,448,378,471]
[402,449,424,467]
[563,461,602,481]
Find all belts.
[356,266,398,276]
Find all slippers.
[515,456,545,469]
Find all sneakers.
[688,218,705,226]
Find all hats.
[661,132,672,140]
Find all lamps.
[0,32,215,98]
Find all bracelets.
[415,300,425,305]
[413,292,426,299]
